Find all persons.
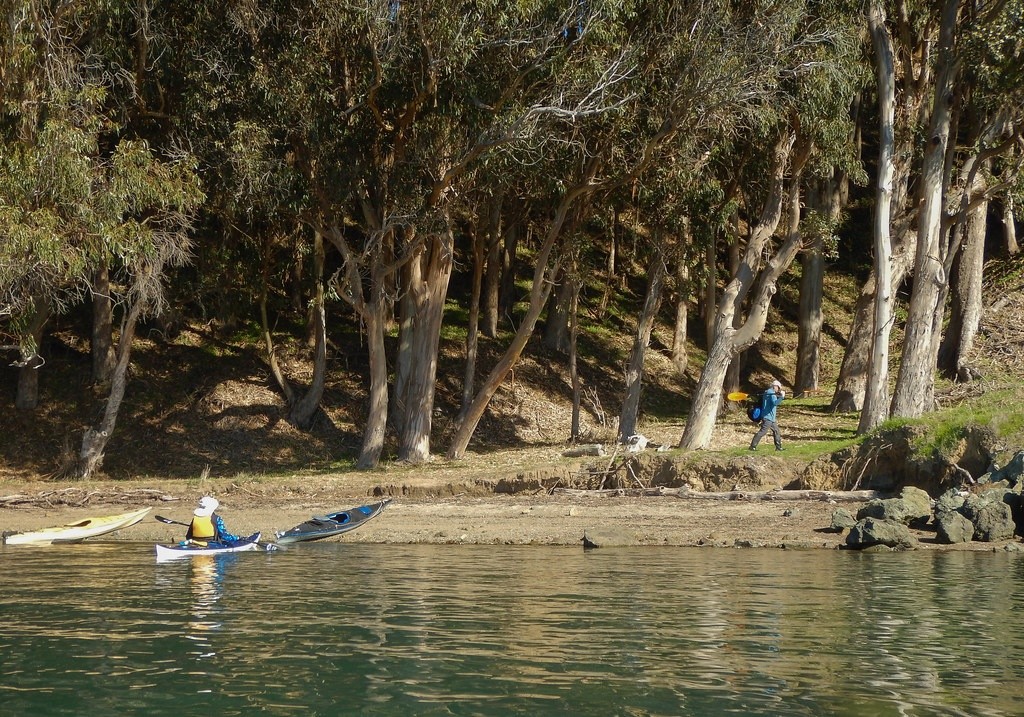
[748,380,786,451]
[185,496,244,545]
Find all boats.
[5,507,153,549]
[276,499,395,553]
[155,531,262,574]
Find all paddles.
[727,390,826,401]
[155,515,287,552]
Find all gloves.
[781,391,785,397]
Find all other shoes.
[775,446,787,451]
[749,446,760,451]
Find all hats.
[770,380,782,390]
[193,497,218,517]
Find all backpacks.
[747,398,763,422]
